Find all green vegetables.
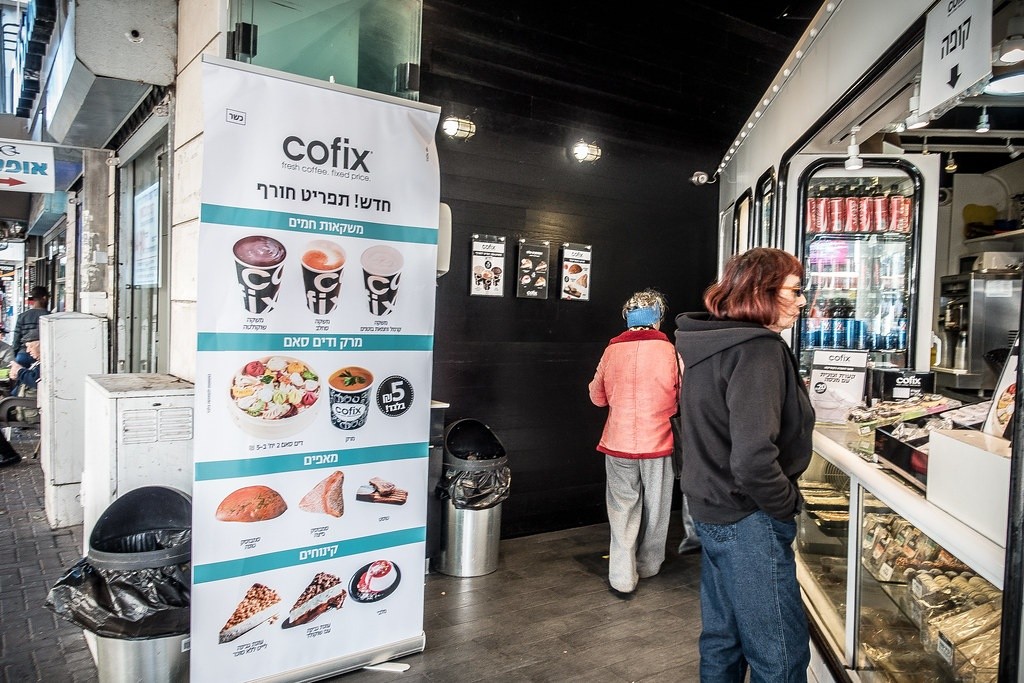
[338,370,366,386]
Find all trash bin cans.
[88,483,192,683]
[437,418,512,580]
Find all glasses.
[766,284,807,297]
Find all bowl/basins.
[225,355,323,439]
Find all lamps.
[945,153,957,174]
[999,38,1024,63]
[844,133,863,170]
[688,170,717,185]
[566,138,602,166]
[1005,144,1022,159]
[975,107,990,133]
[905,84,930,130]
[437,113,477,143]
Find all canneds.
[800,254,908,351]
[805,195,911,234]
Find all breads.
[298,470,344,517]
[576,273,588,288]
[568,264,582,274]
[861,512,1002,683]
[216,485,288,522]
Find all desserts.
[521,259,546,286]
[364,560,397,591]
[218,583,283,644]
[289,571,343,624]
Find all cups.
[301,239,347,314]
[233,236,288,314]
[473,266,502,290]
[327,366,375,430]
[361,245,404,316]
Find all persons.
[13,286,54,360]
[588,287,685,600]
[0,328,14,394]
[674,248,816,683]
[0,327,40,468]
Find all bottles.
[798,177,917,352]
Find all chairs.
[0,386,41,459]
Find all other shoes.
[0,454,22,468]
[609,586,636,599]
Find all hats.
[21,329,38,342]
